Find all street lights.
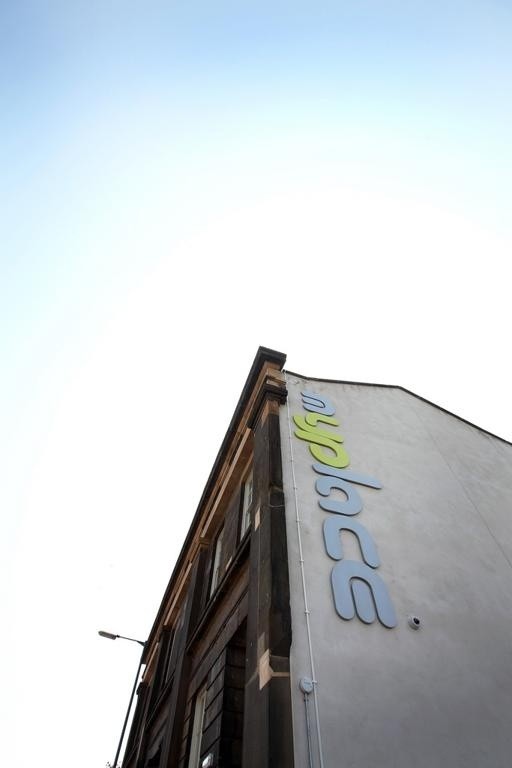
[96,625,147,766]
